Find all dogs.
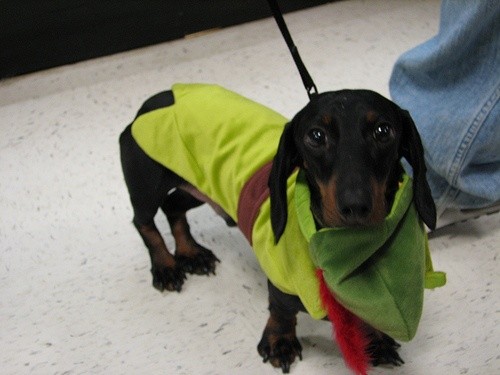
[118,83,438,375]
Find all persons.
[386,0,499,239]
